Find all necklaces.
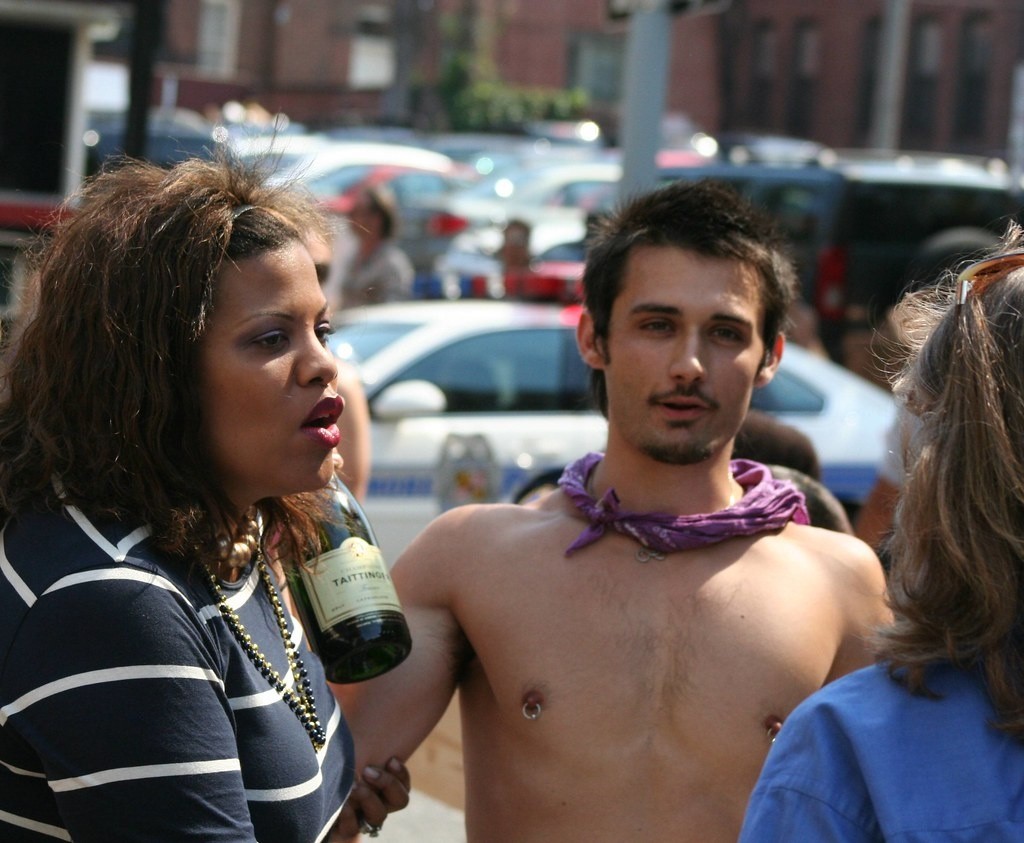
[203,508,264,567]
[198,548,327,749]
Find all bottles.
[278,472,412,685]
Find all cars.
[0,118,1024,407]
[311,295,921,579]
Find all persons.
[328,142,1024,843]
[0,150,410,843]
